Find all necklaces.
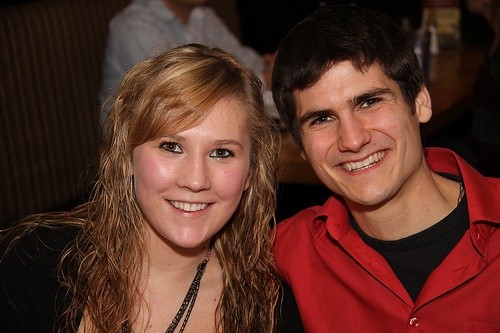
[119,241,214,333]
[456,178,466,205]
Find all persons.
[263,6,500,332]
[99,0,275,151]
[0,44,307,333]
